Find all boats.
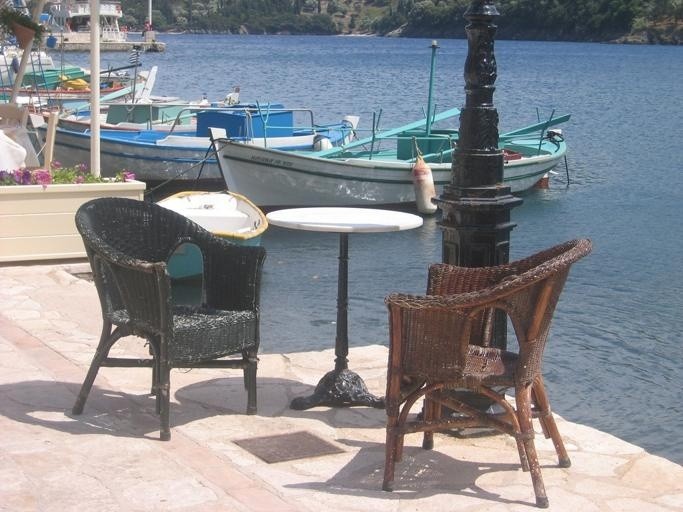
[0,65,159,107]
[44,86,240,129]
[217,37,572,206]
[37,102,360,203]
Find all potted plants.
[0,7,48,57]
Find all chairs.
[68,197,266,441]
[382,237,593,509]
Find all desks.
[266,206,423,410]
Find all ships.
[0,0,125,41]
